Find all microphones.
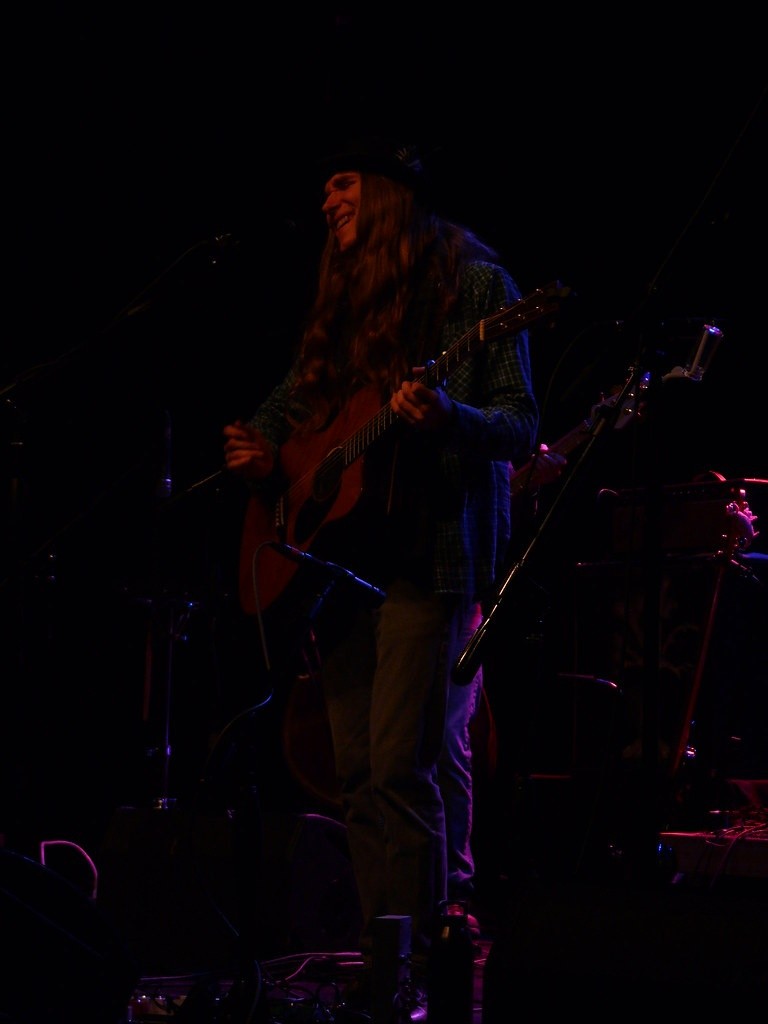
[215,220,296,249]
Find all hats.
[320,138,410,183]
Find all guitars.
[511,374,653,502]
[238,286,572,624]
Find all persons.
[222,136,542,1024]
